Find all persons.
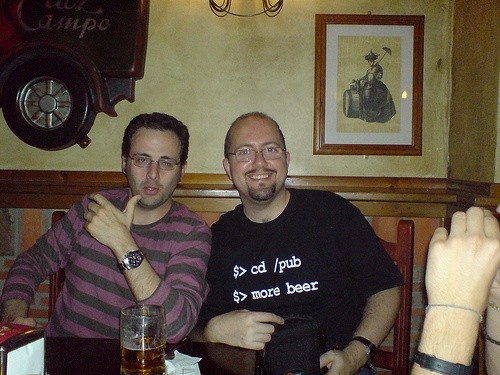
[187,112,406,375]
[0,112,213,344]
[409,204,500,375]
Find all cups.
[120,304,166,375]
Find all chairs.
[370,218,415,375]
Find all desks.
[45,337,263,375]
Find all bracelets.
[426,304,483,323]
[413,351,474,375]
[484,330,500,346]
[487,302,500,312]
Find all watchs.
[118,249,144,271]
[351,337,376,361]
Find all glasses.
[128,156,182,170]
[227,146,286,162]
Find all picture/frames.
[315,15,423,156]
